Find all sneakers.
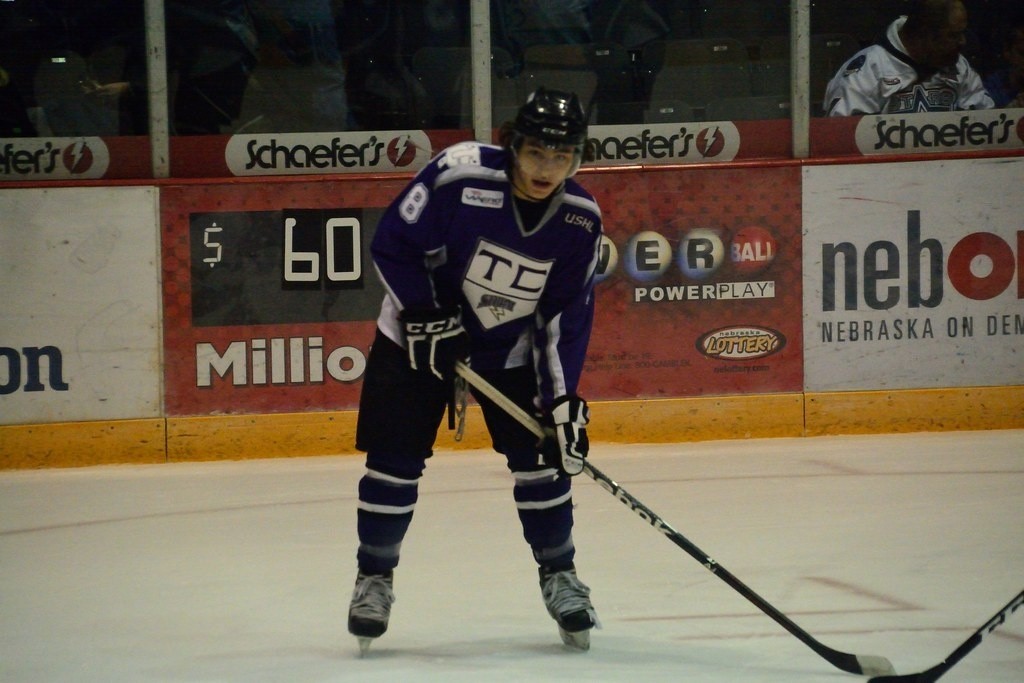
[539,566,596,651]
[348,566,394,657]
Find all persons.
[822,1,1023,118]
[347,86,604,657]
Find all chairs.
[0,0,1024,138]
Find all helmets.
[516,87,587,145]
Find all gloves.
[536,394,590,476]
[395,303,471,381]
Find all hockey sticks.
[865,587,1024,683]
[449,355,899,680]
